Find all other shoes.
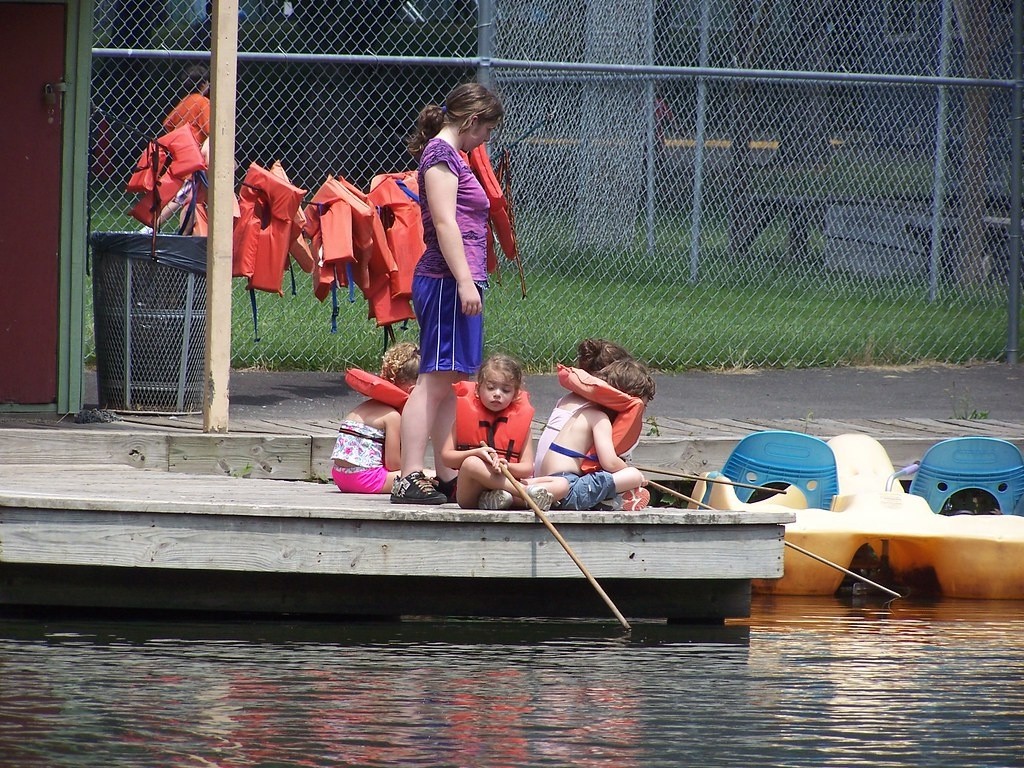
[477,487,515,511]
[587,485,651,512]
[525,485,554,512]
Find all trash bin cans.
[93,231,211,416]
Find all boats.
[686,431,1024,601]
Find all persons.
[139,67,209,236]
[642,98,675,153]
[533,337,656,510]
[442,355,569,512]
[390,82,505,505]
[331,342,436,494]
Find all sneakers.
[390,470,449,504]
[428,474,459,503]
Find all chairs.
[696,422,1024,517]
